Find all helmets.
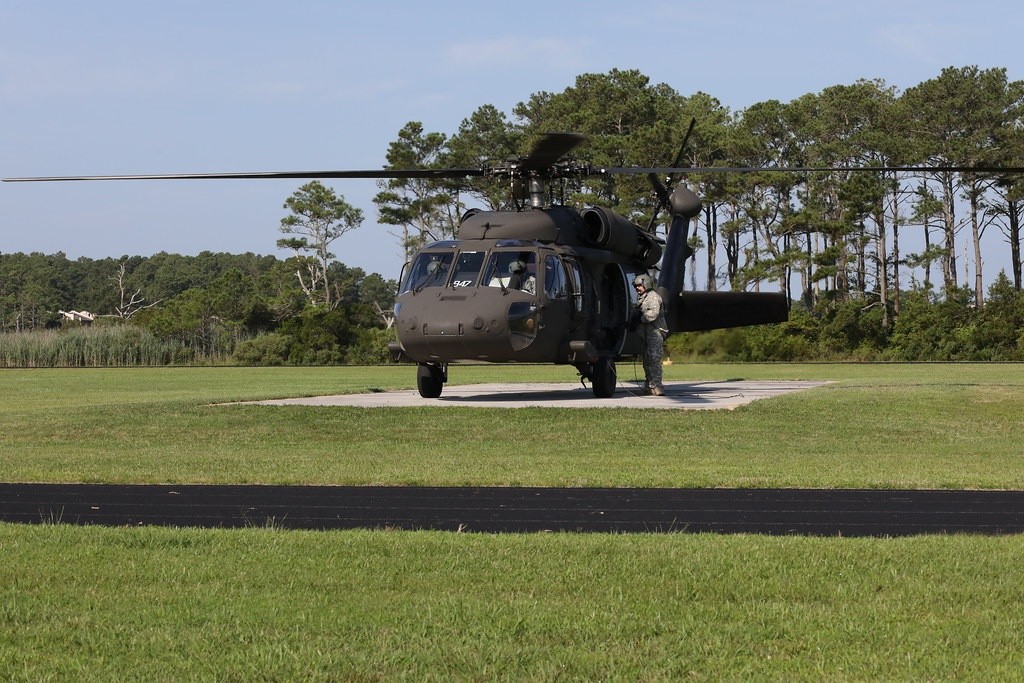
[427,262,447,274]
[508,259,528,275]
[632,275,652,291]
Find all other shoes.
[637,387,654,395]
[654,385,665,395]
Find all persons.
[630,273,668,396]
[508,261,549,299]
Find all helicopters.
[0,118,1024,397]
